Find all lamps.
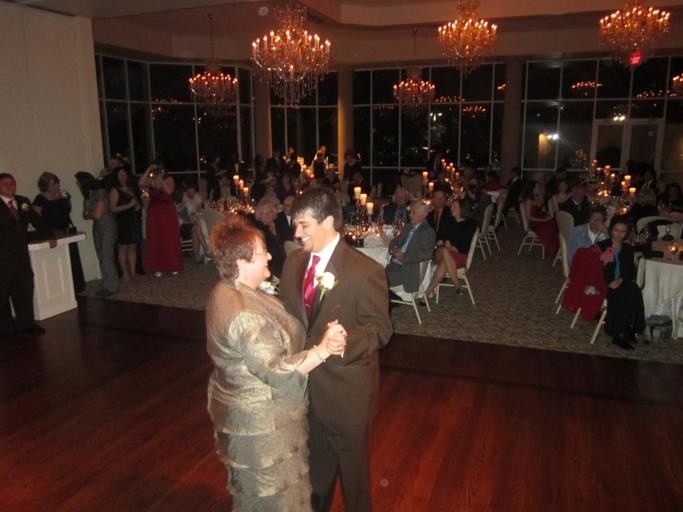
[435,95,465,104]
[637,88,677,101]
[597,0,673,53]
[461,103,486,119]
[251,0,333,107]
[187,13,239,100]
[570,80,605,95]
[435,0,500,62]
[610,110,626,123]
[391,27,436,103]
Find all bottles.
[354,209,383,233]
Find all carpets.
[84,219,682,364]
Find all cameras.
[149,168,163,178]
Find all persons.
[201,211,349,512]
[526,159,682,350]
[181,146,368,272]
[2,171,85,344]
[75,155,183,299]
[380,151,526,304]
[276,187,395,512]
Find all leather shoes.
[3,325,47,335]
[612,337,636,351]
[419,296,429,307]
[455,287,465,296]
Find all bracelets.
[312,343,327,364]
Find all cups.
[355,239,363,248]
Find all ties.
[302,255,320,321]
[8,199,20,224]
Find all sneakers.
[95,286,118,299]
[122,275,139,283]
[194,252,213,265]
[154,269,179,277]
